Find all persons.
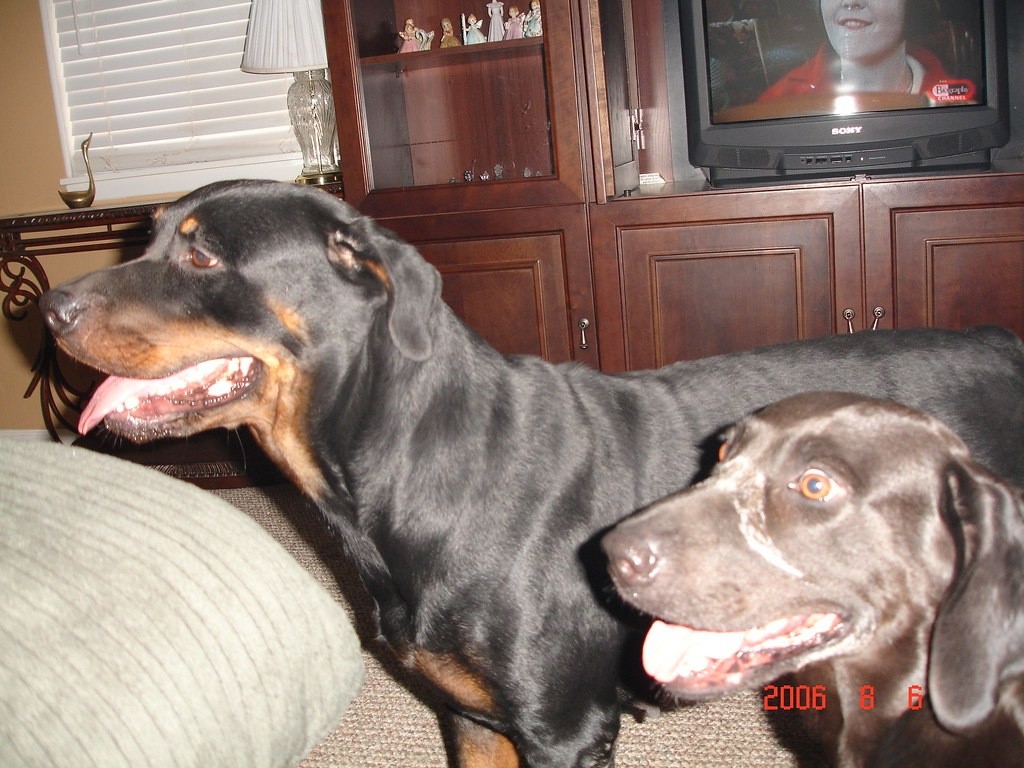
[440,18,460,48]
[758,1,960,100]
[486,0,504,42]
[501,7,525,40]
[525,0,542,37]
[462,15,487,45]
[399,19,422,53]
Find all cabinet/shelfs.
[370,202,600,373]
[319,0,596,219]
[590,174,1024,372]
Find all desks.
[0,199,265,489]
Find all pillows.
[0,446,364,768]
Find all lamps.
[240,0,341,187]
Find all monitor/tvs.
[677,0,1009,191]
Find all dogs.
[597,394,1024,768]
[34,174,1024,768]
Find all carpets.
[203,482,795,768]
